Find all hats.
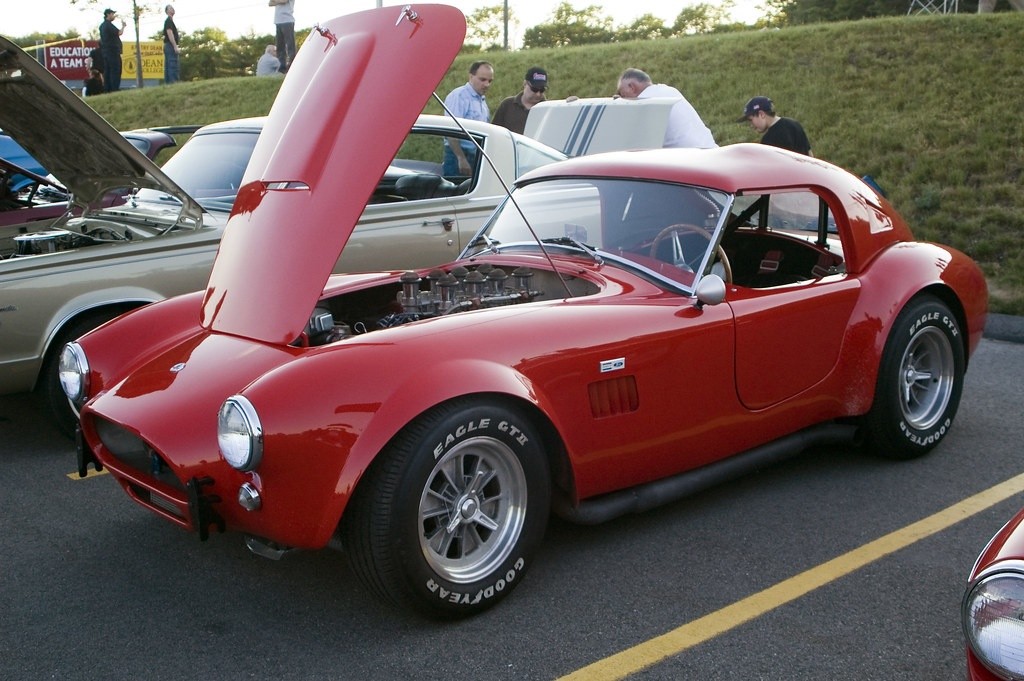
[525,67,548,88]
[735,96,774,123]
[104,9,117,15]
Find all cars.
[58,0,988,621]
[0,35,685,439]
[0,125,207,255]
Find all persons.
[443,60,494,185]
[81,38,104,96]
[491,66,550,134]
[255,44,280,75]
[268,0,297,72]
[747,96,814,157]
[162,4,183,84]
[616,66,720,149]
[99,7,126,92]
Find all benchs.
[640,228,844,287]
[394,173,472,200]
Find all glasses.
[527,82,546,93]
